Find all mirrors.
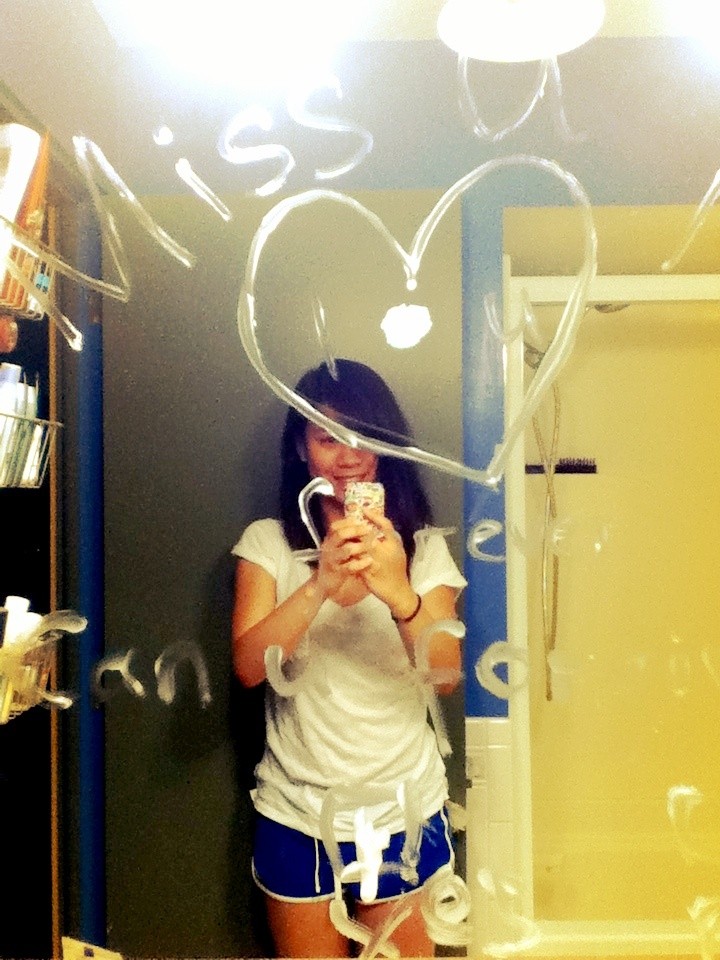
[0,0,720,960]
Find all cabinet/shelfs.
[0,82,106,960]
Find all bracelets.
[391,595,421,623]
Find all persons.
[229,358,467,960]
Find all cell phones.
[345,483,385,540]
